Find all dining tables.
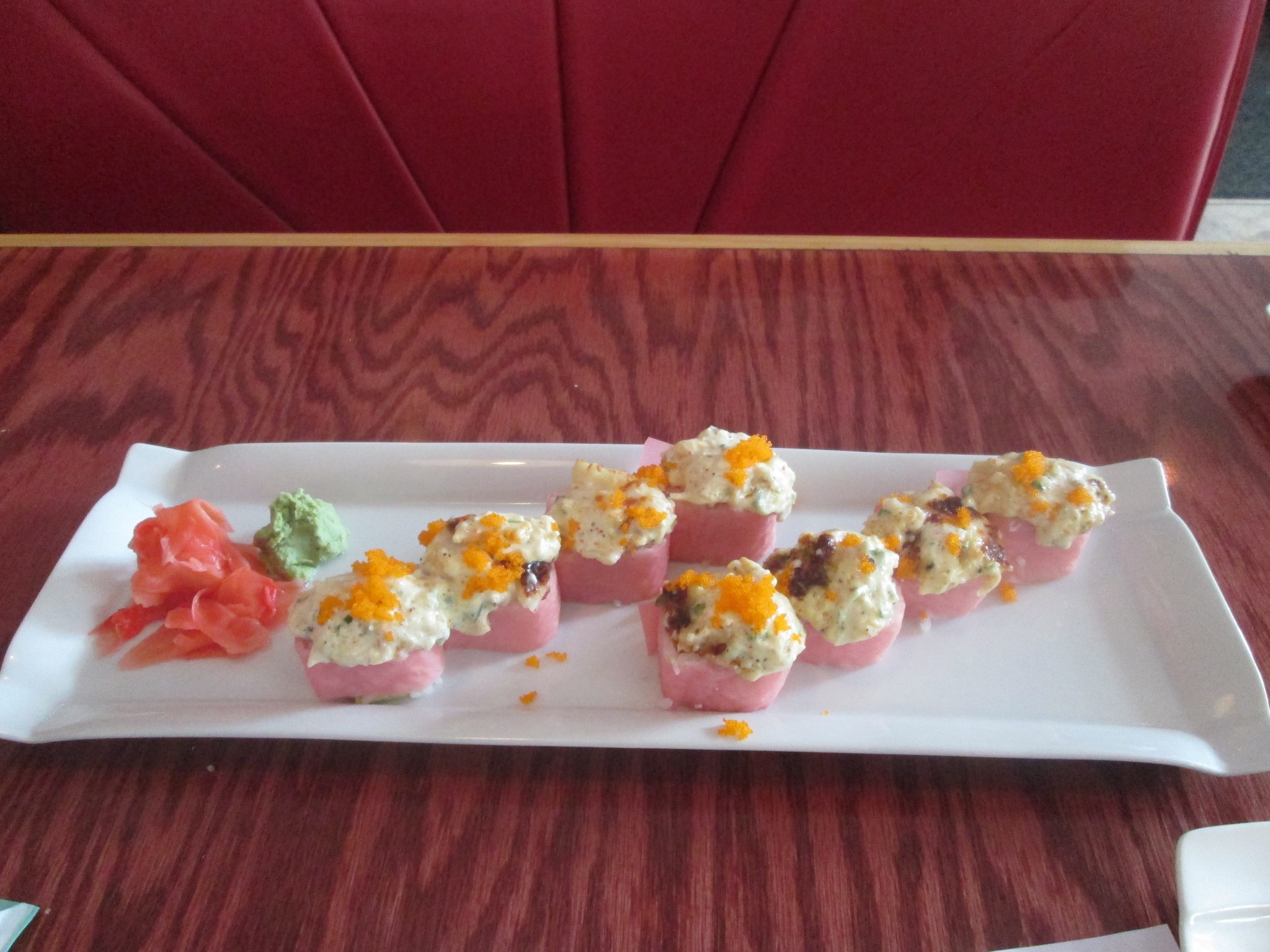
[0,235,1270,952]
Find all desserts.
[287,426,1115,713]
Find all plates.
[1,442,1270,775]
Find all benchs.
[0,0,1265,238]
